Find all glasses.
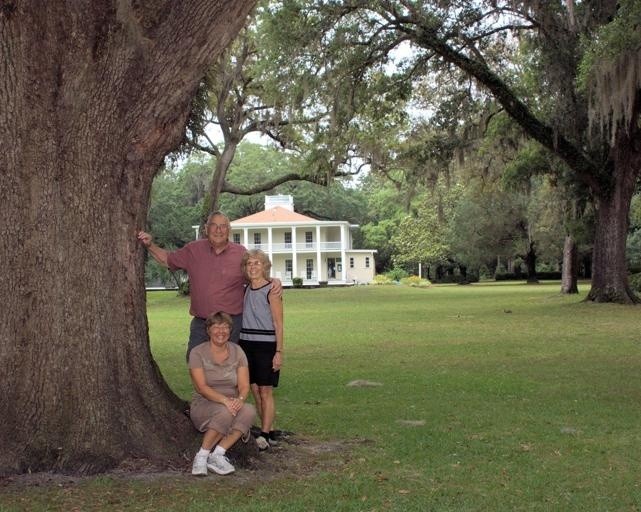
[210,326,231,332]
[209,223,227,231]
[246,261,262,266]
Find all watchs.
[238,396,245,401]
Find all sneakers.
[191,454,236,476]
[255,431,279,451]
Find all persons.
[188,311,257,476]
[237,249,284,451]
[137,209,283,416]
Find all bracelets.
[275,350,280,353]
